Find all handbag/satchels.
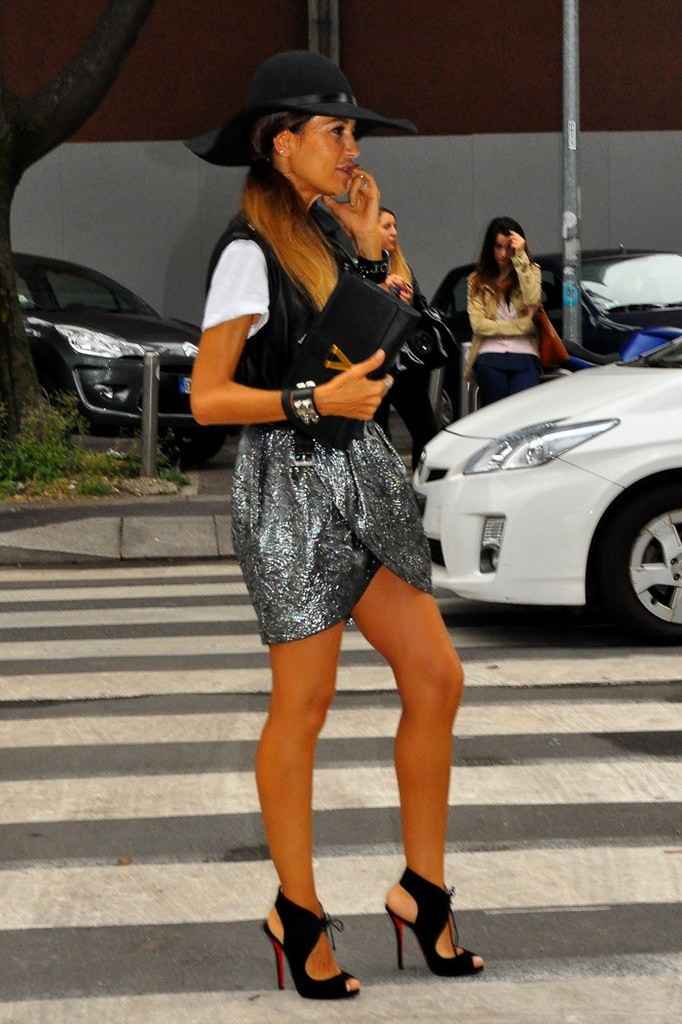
[285,275,420,448]
[409,286,460,369]
[535,302,571,371]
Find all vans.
[431,244,682,427]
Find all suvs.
[9,250,241,466]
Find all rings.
[360,184,368,191]
[358,174,366,179]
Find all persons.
[181,51,488,1000]
[370,205,440,471]
[458,216,543,408]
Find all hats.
[181,48,420,169]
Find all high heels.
[263,884,362,1000]
[383,866,484,977]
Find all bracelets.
[358,247,390,285]
[282,389,302,428]
[294,381,321,425]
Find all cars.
[410,331,682,644]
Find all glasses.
[495,242,514,252]
